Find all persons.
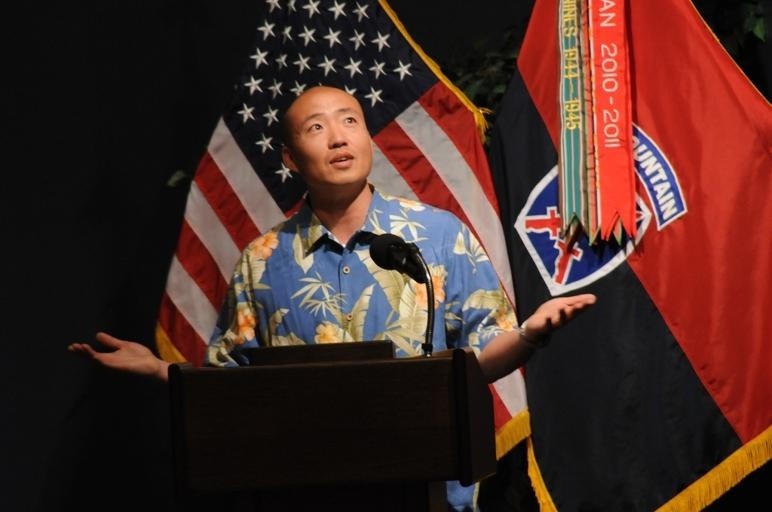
[66,85,597,511]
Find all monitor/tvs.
[235,337,397,364]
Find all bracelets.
[516,319,541,351]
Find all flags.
[154,0,530,461]
[486,0,772,511]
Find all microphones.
[370,234,439,357]
